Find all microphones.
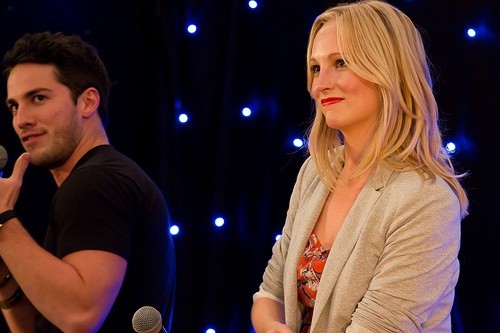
[0,145,8,178]
[132,305,168,333]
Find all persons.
[0,29,180,333]
[250,1,469,333]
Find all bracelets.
[0,286,26,310]
[0,271,12,288]
[0,208,16,228]
